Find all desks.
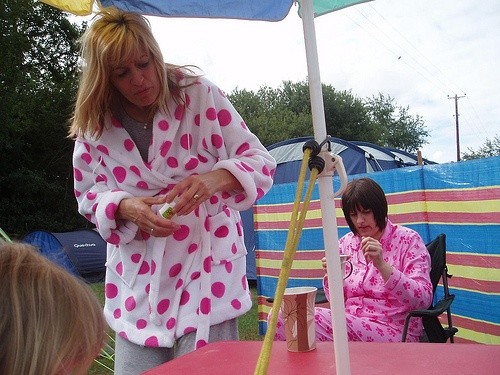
[137,340,500,375]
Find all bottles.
[159,200,177,222]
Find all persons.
[64,6,277,346]
[266,177,433,343]
[0,240,106,375]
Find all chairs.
[402,233,458,343]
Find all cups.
[339,254,353,288]
[282,286,318,353]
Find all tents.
[24,228,105,285]
[240,135,438,281]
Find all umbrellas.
[42,0,364,375]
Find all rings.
[193,194,200,200]
[150,229,154,234]
